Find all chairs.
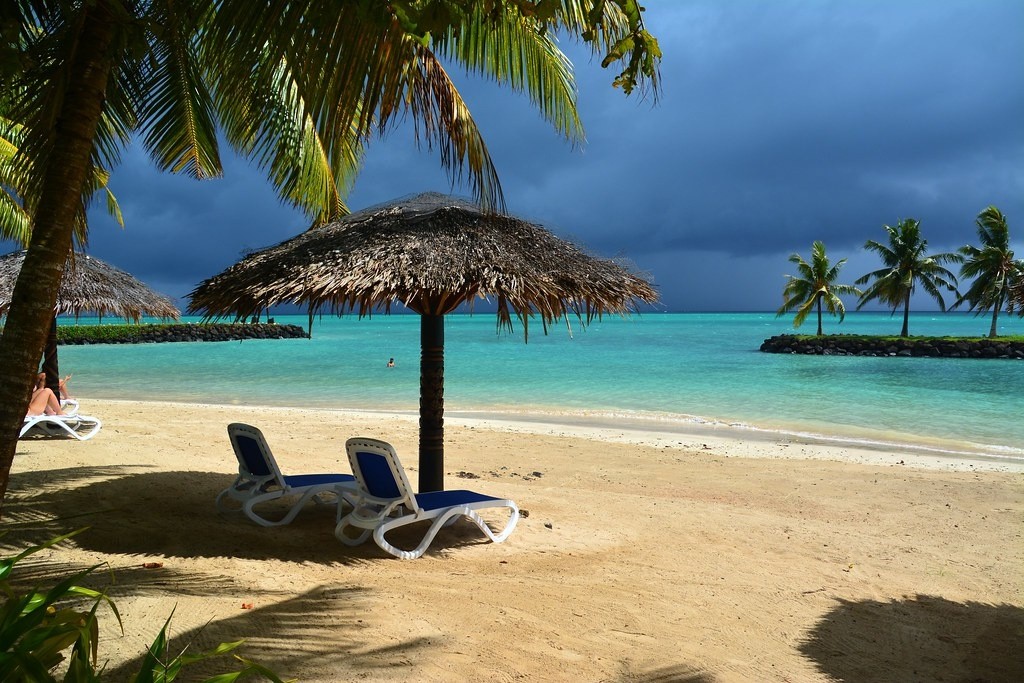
[60,400,80,415]
[335,437,518,560]
[213,423,356,528]
[19,416,101,441]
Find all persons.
[25,361,73,416]
[387,358,394,367]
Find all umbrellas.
[183,193,658,494]
[0,248,180,428]
